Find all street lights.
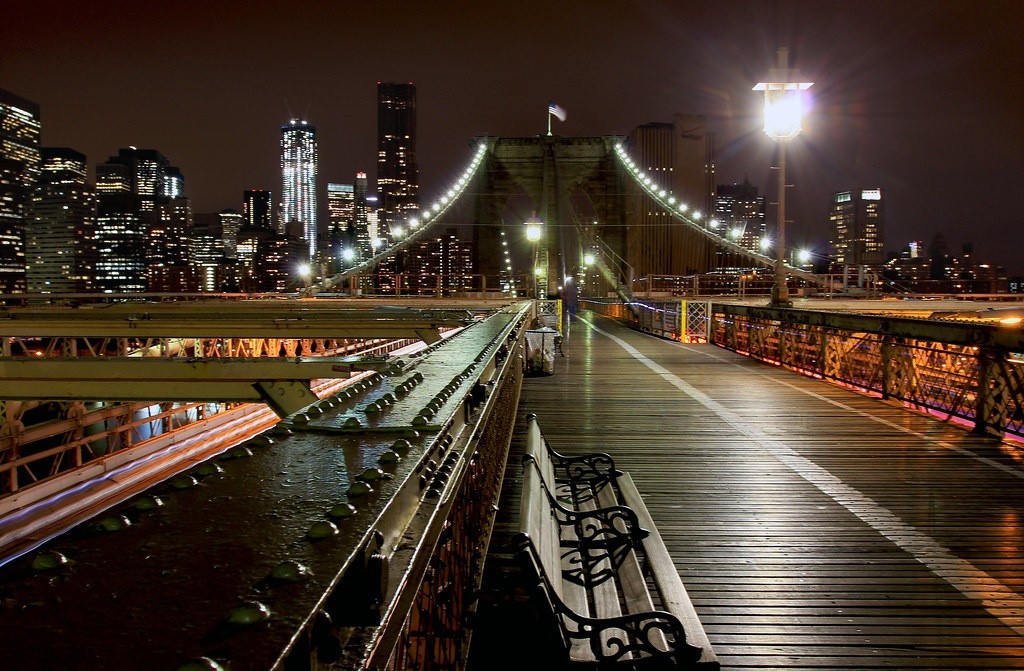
[524,210,544,297]
[752,46,815,307]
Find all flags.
[548,101,568,123]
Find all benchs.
[512,412,720,670]
[537,319,565,358]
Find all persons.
[560,280,579,324]
